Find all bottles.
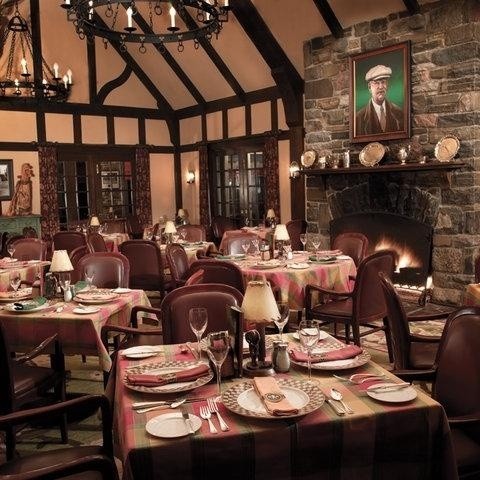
[365,65,392,82]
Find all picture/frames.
[349,40,411,142]
[0,159,14,200]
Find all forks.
[200,404,218,434]
[206,396,229,432]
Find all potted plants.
[349,40,411,142]
[0,159,14,200]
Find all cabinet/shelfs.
[287,248,293,259]
[271,342,291,375]
[64,286,72,302]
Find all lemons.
[396,144,412,166]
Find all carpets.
[380,105,386,133]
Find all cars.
[416,155,430,164]
[319,151,351,169]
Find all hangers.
[136,397,187,415]
[328,386,354,416]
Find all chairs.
[0,216,480,479]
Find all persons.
[355,64,408,138]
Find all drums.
[178,404,196,435]
[355,382,412,392]
[132,397,206,409]
[120,351,167,355]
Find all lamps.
[266,209,275,222]
[62,0,233,53]
[178,209,186,223]
[187,170,194,184]
[0,0,74,105]
[290,161,300,182]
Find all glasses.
[187,170,194,184]
[0,0,74,105]
[62,0,233,53]
[290,161,300,182]
[266,209,275,222]
[178,209,186,223]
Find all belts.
[297,320,321,385]
[189,309,209,362]
[146,229,190,246]
[208,329,231,403]
[9,268,96,305]
[240,233,322,268]
[7,243,17,261]
[271,301,292,341]
[74,222,109,236]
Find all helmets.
[199,331,275,355]
[0,286,132,313]
[365,383,418,403]
[301,149,318,169]
[123,361,215,393]
[309,249,343,264]
[222,376,327,420]
[358,142,386,167]
[252,260,309,269]
[144,411,203,439]
[287,343,372,370]
[123,345,160,358]
[434,135,461,162]
